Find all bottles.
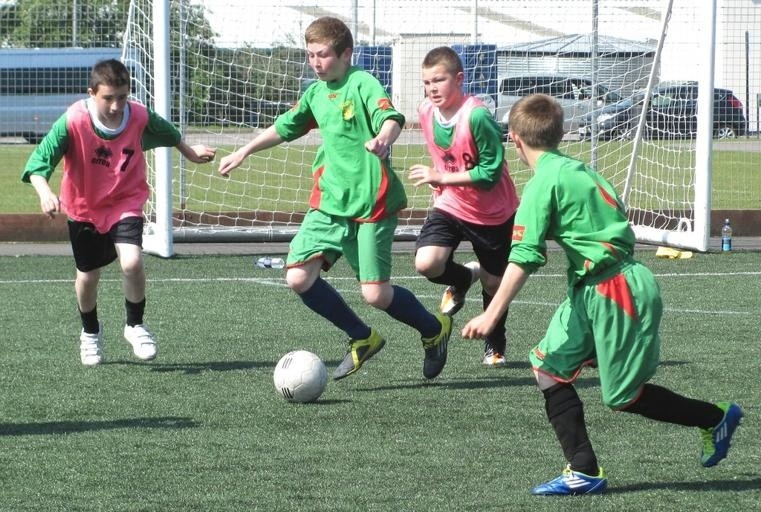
[254,257,284,269]
[721,219,732,251]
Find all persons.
[408,47,518,364]
[22,60,216,366]
[462,92,744,495]
[218,17,453,381]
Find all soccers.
[274,350,327,403]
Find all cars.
[577,84,745,141]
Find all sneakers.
[124,323,157,361]
[441,261,480,315]
[80,319,104,365]
[333,327,386,381]
[483,341,509,368]
[532,463,607,496]
[421,314,453,379]
[697,401,744,467]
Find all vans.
[494,72,626,141]
[0,47,141,144]
